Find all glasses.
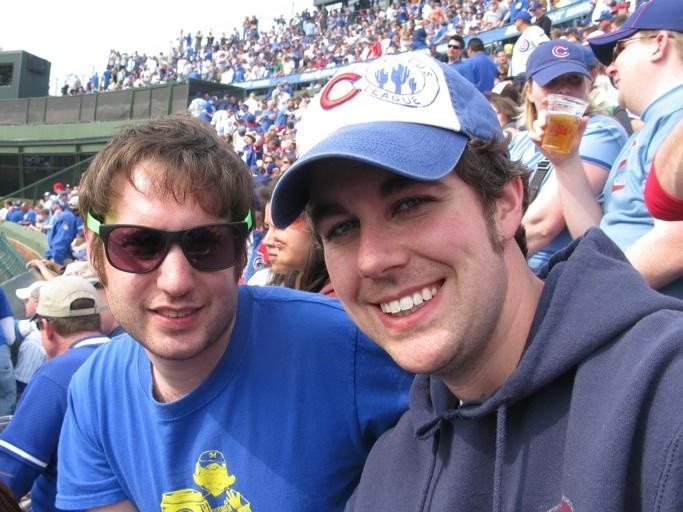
[448,44,460,50]
[86,212,253,274]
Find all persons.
[53,112,416,512]
[270,54,681,511]
[0,277,110,512]
[0,183,124,414]
[63,1,681,416]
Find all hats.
[269,51,509,234]
[197,93,260,144]
[610,3,629,16]
[13,182,87,235]
[594,10,612,23]
[528,0,543,12]
[15,280,49,302]
[586,1,683,66]
[29,274,99,323]
[526,37,593,88]
[511,11,532,23]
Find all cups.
[540,94,589,156]
[503,41,517,57]
